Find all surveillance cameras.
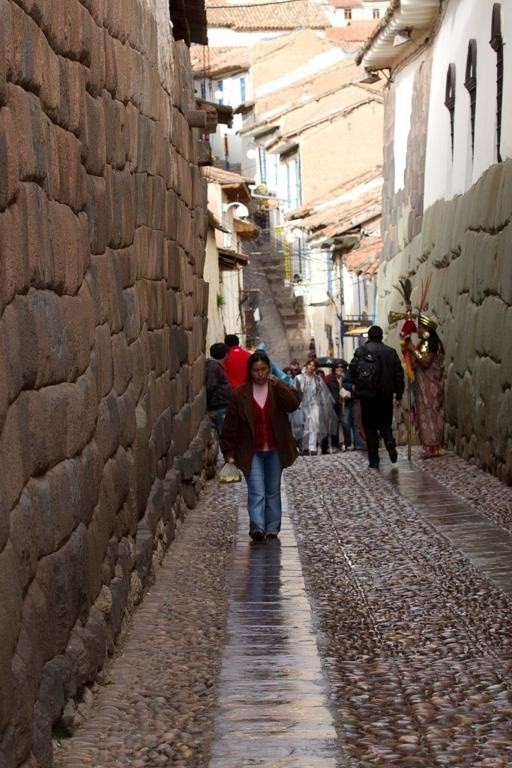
[237,206,250,222]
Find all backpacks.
[351,343,376,393]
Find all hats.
[419,314,437,331]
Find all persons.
[290,274,306,315]
[247,302,263,336]
[219,351,302,542]
[205,314,445,467]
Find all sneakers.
[297,444,363,457]
[368,448,398,468]
[248,525,280,542]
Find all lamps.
[224,202,249,220]
